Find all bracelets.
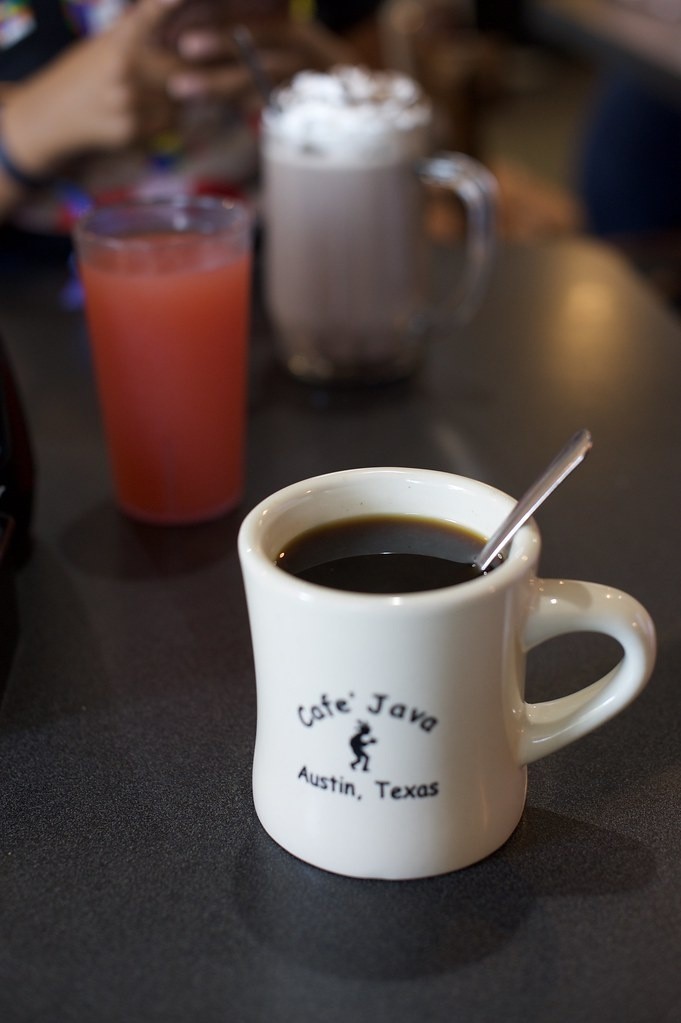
[1,150,60,191]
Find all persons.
[0,0,290,232]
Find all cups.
[258,101,499,385]
[236,467,656,881]
[69,193,254,525]
